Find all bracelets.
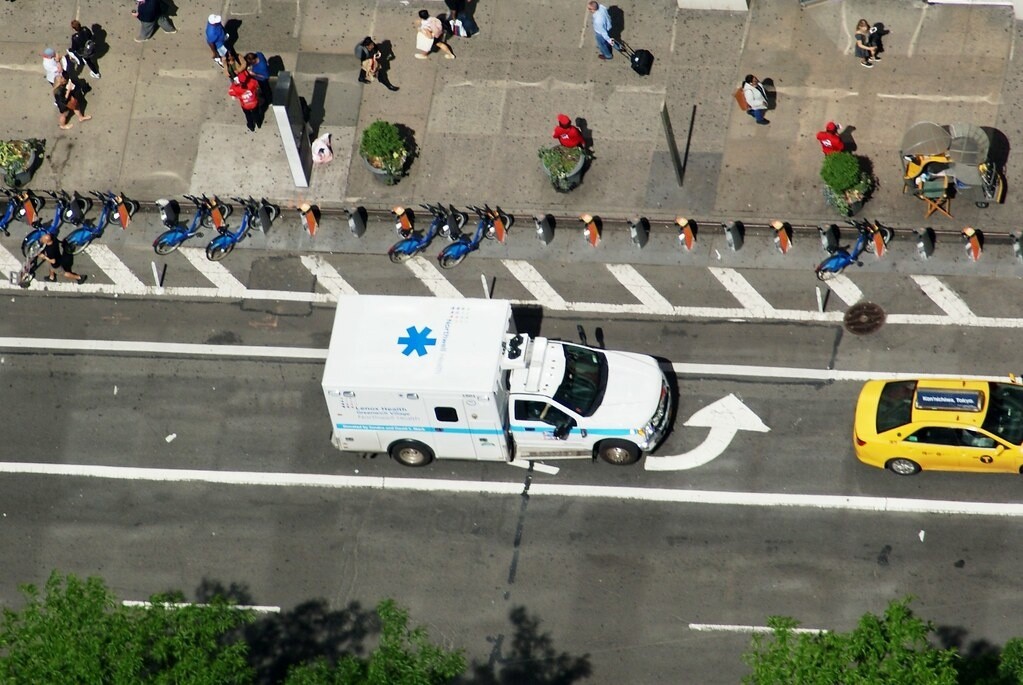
[56,61,59,62]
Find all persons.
[816,122,844,155]
[206,15,225,67]
[589,1,614,59]
[67,20,101,79]
[41,49,63,106]
[855,19,881,68]
[552,114,586,149]
[134,0,177,42]
[742,75,778,124]
[222,49,267,131]
[358,37,398,90]
[445,0,480,34]
[53,76,91,129]
[415,10,456,59]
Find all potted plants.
[360,119,409,187]
[537,143,594,194]
[819,150,872,218]
[1,138,44,188]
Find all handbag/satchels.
[735,88,755,111]
[65,96,78,109]
[449,19,467,37]
[416,31,434,51]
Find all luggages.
[612,39,654,76]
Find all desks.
[911,175,954,220]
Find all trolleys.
[8,242,48,289]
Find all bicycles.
[435,203,512,271]
[63,191,140,260]
[387,199,469,263]
[203,195,280,261]
[20,191,90,258]
[815,217,894,281]
[0,186,44,238]
[152,195,232,255]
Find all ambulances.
[319,294,676,469]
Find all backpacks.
[75,29,94,57]
[425,17,442,38]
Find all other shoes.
[598,54,612,59]
[868,56,881,62]
[388,85,399,91]
[445,54,455,59]
[90,71,100,79]
[59,124,72,130]
[134,36,149,43]
[358,78,370,84]
[860,60,873,68]
[164,30,177,34]
[415,53,427,59]
[78,115,92,122]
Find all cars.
[852,372,1023,475]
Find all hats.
[826,122,839,132]
[238,70,249,82]
[208,14,221,24]
[43,49,55,58]
